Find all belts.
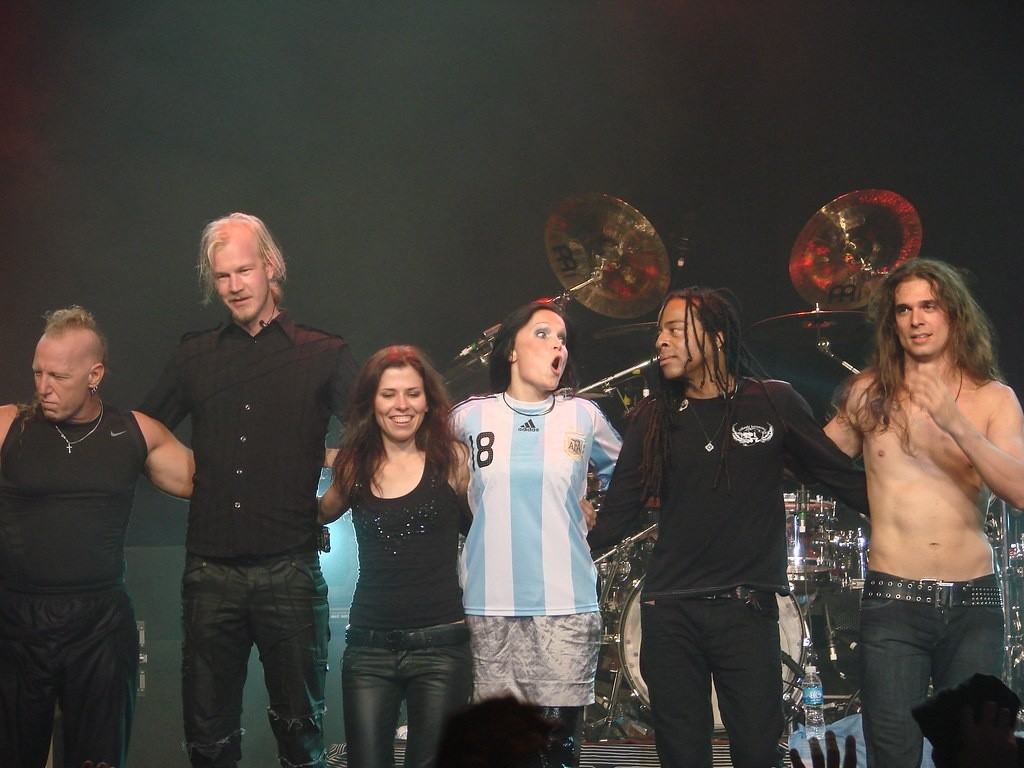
[692,587,771,610]
[345,623,470,649]
[862,574,1003,606]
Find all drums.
[615,560,809,719]
[782,483,872,591]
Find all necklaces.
[53,398,103,453]
[502,391,556,416]
[902,363,962,403]
[684,380,737,452]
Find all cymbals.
[789,187,922,311]
[545,192,672,319]
[747,310,879,347]
[592,322,660,342]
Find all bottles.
[801,665,825,740]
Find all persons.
[322,346,597,768]
[589,287,997,768]
[0,306,195,768]
[436,695,579,768]
[781,259,1024,768]
[141,213,365,768]
[789,673,1023,768]
[324,303,624,768]
